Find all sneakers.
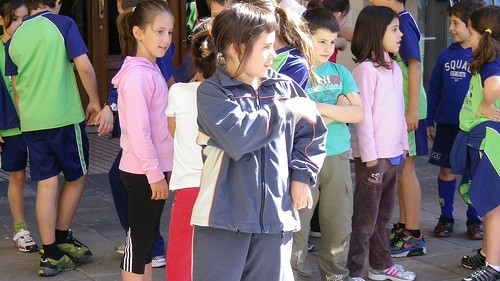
[461,262,500,281]
[343,275,366,281]
[38,249,82,277]
[461,248,486,270]
[12,228,39,252]
[467,223,483,240]
[434,215,455,237]
[38,230,93,262]
[388,222,406,240]
[367,259,416,281]
[388,229,426,258]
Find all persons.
[291,7,364,281]
[208,0,355,252]
[0,0,39,253]
[120,1,175,281]
[5,0,102,275]
[370,0,427,258]
[190,3,328,281]
[165,30,217,281]
[427,0,484,240]
[346,6,416,281]
[450,5,500,281]
[95,1,176,267]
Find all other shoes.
[152,254,166,268]
[309,229,321,237]
[115,242,125,255]
[308,239,315,251]
